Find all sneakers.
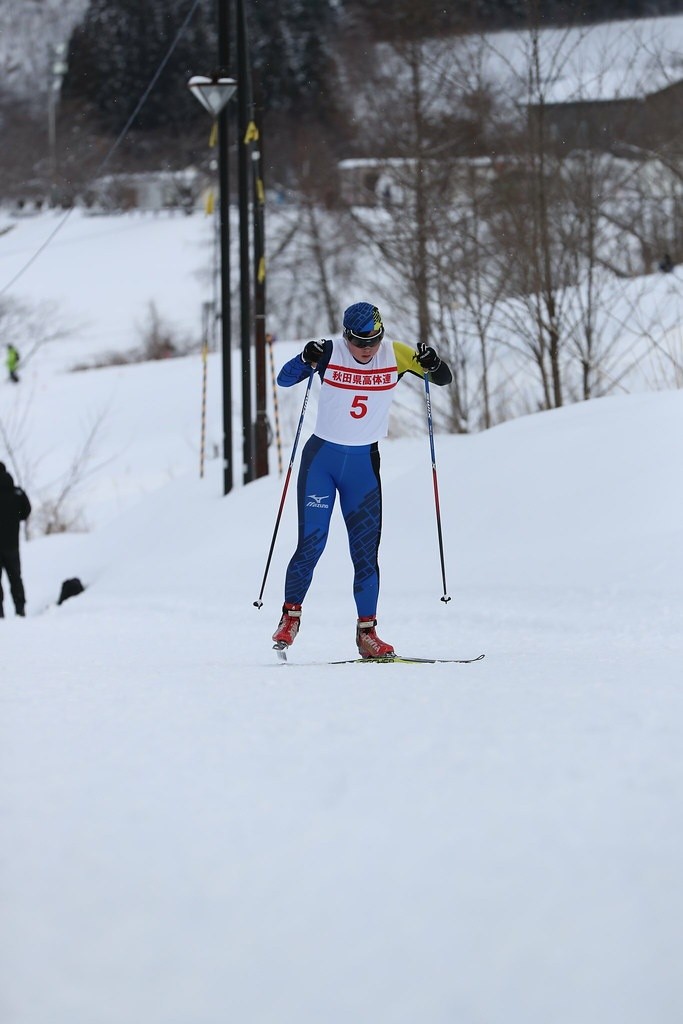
[272,603,302,646]
[355,617,394,656]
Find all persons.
[0,462,31,618]
[272,302,452,657]
[5,345,19,382]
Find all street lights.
[186,73,239,497]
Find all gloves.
[300,341,324,364]
[413,347,440,372]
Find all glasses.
[345,327,383,348]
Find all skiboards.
[260,649,487,667]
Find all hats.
[343,302,384,331]
